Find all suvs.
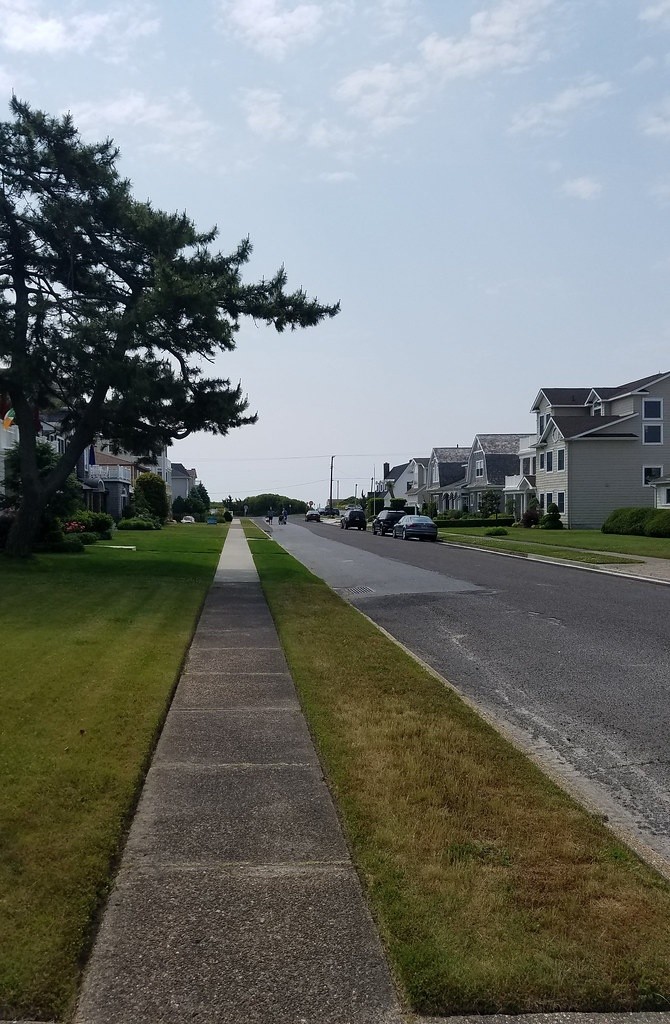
[372,510,407,537]
[339,510,367,531]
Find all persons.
[268,507,274,525]
[282,507,288,524]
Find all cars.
[305,510,320,522]
[180,515,195,524]
[393,514,439,543]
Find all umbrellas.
[3,408,15,430]
